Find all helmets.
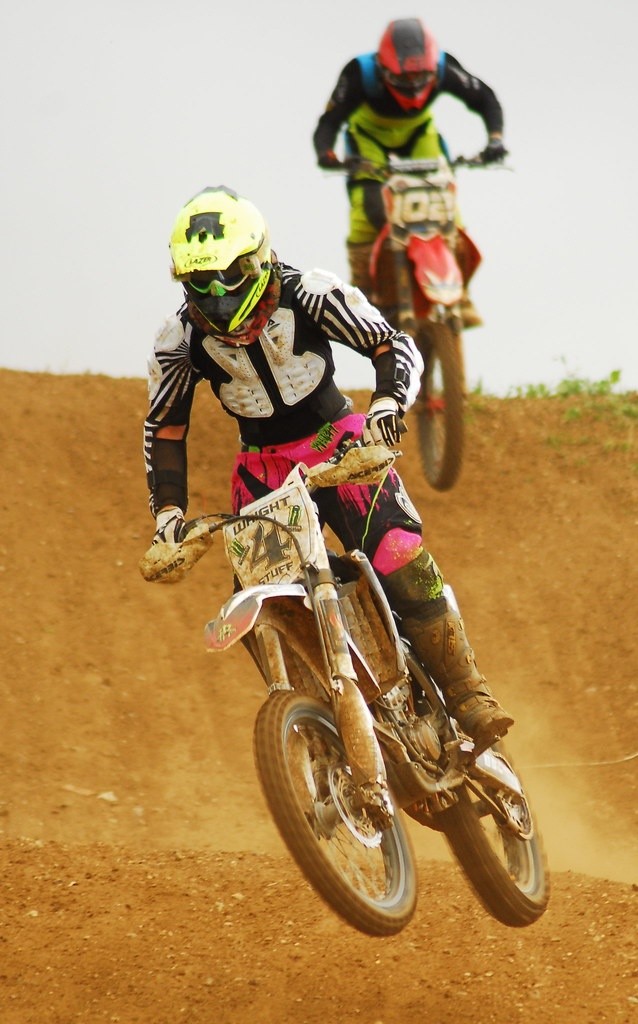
[380,20,437,112]
[169,186,283,346]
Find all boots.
[402,612,514,743]
[243,628,330,806]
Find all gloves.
[151,505,186,547]
[361,394,401,450]
[317,149,342,169]
[483,137,508,164]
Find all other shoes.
[460,289,479,326]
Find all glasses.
[383,68,437,89]
[173,251,262,295]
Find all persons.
[144,189,514,740]
[314,19,508,329]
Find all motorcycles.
[336,151,516,492]
[139,418,550,938]
